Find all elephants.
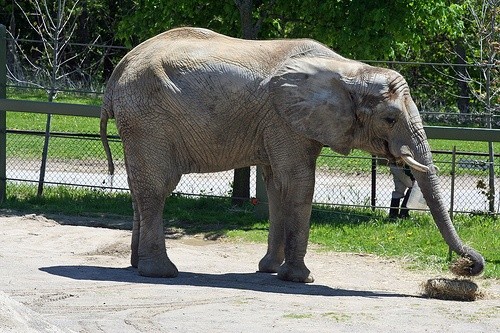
[99,27,485,283]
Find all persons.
[386,158,417,222]
